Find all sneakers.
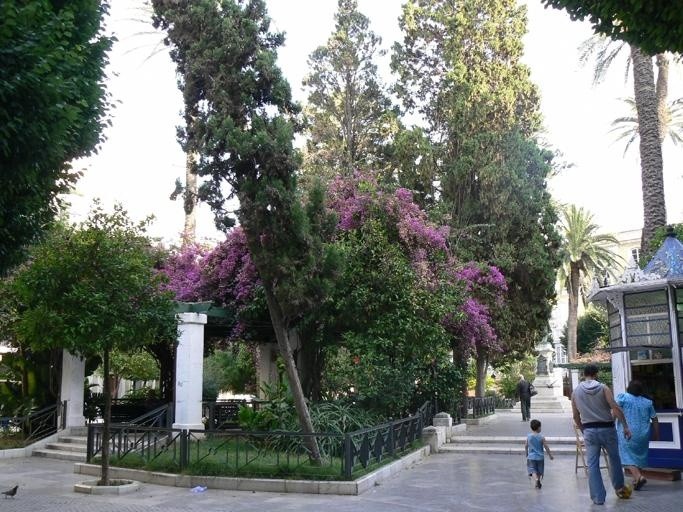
[535,480,542,488]
[616,487,624,498]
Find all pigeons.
[0,484,19,501]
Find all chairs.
[573,424,610,475]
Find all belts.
[582,420,615,429]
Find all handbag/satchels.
[528,383,538,396]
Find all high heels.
[632,476,646,490]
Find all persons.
[616,379,662,490]
[516,373,535,422]
[525,420,554,489]
[572,364,632,505]
